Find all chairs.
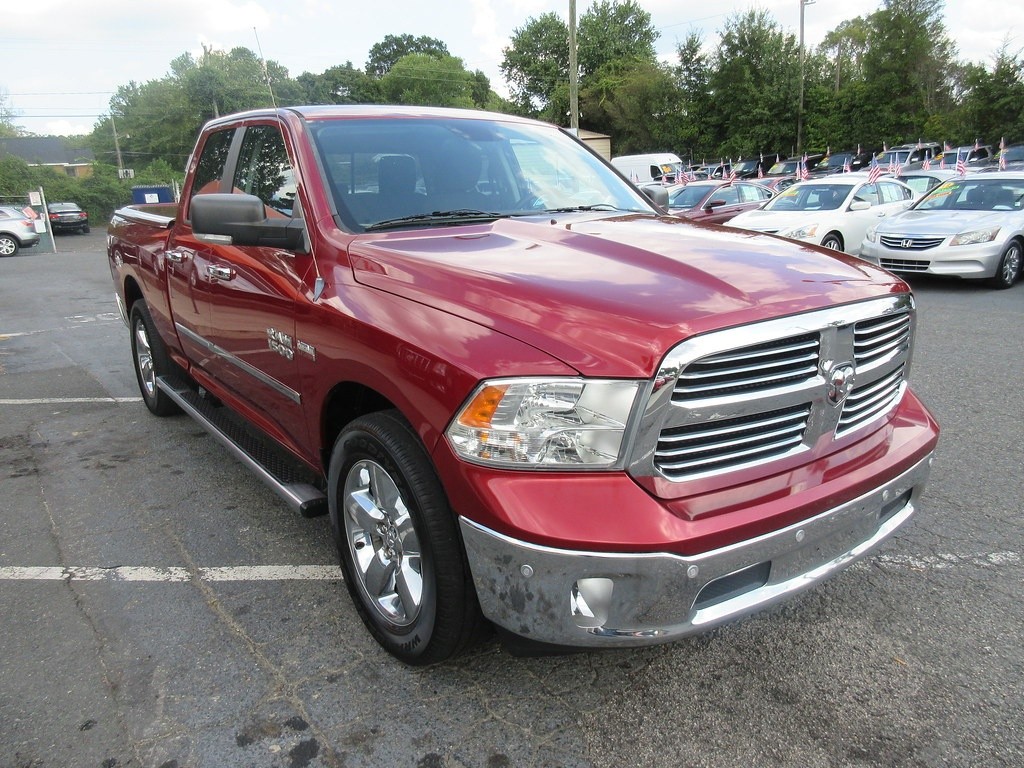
[990,190,1017,210]
[961,188,985,209]
[424,144,493,214]
[818,191,834,210]
[292,161,371,225]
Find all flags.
[974,138,980,152]
[630,144,861,187]
[999,137,1007,170]
[868,138,951,185]
[956,147,966,176]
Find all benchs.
[350,156,429,221]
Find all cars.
[0,207,41,258]
[858,171,1024,289]
[48,202,90,236]
[743,177,930,257]
[634,141,1024,225]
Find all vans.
[610,153,690,186]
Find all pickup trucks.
[107,103,942,669]
